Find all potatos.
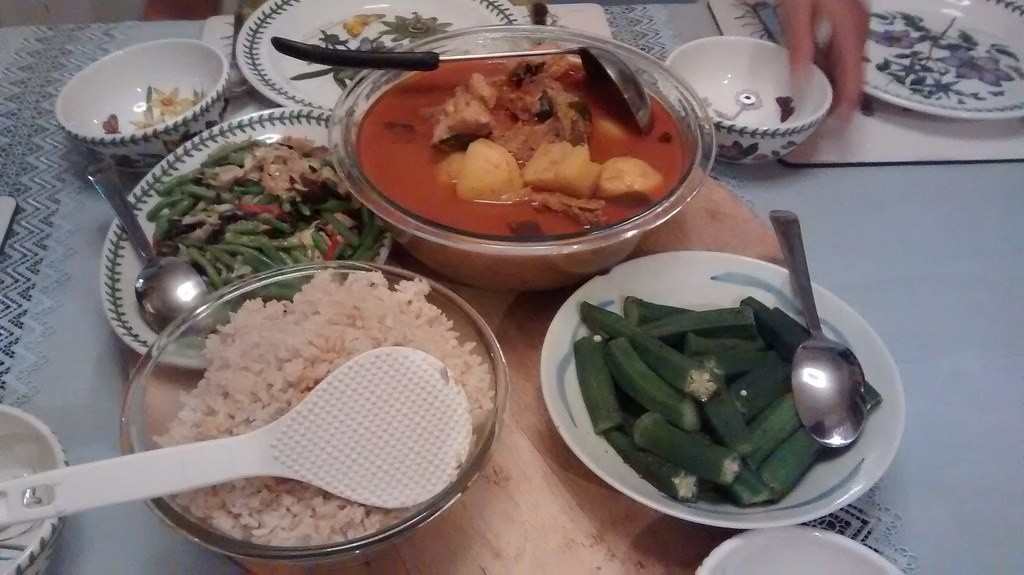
[437,138,666,207]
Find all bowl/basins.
[123,258,509,568]
[326,23,717,292]
[56,38,231,170]
[666,37,832,162]
[696,525,905,574]
[1,404,69,575]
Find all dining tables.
[0,0,1024,575]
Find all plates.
[814,1,1024,120]
[235,0,521,110]
[540,251,905,530]
[95,106,392,372]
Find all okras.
[571,297,885,508]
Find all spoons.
[770,209,866,449]
[86,160,208,337]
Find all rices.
[151,268,493,551]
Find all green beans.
[145,139,386,348]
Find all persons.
[776,0,869,159]
